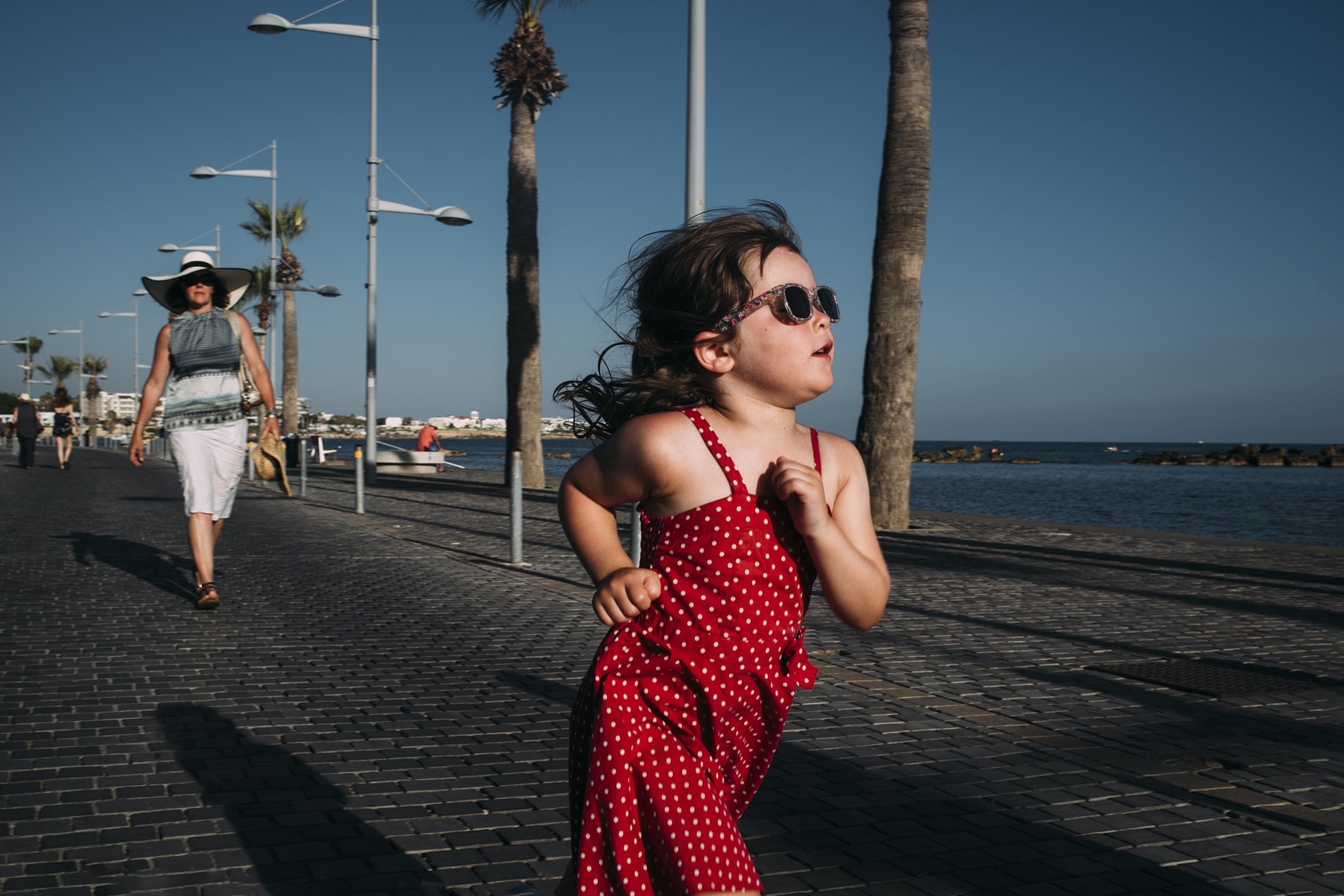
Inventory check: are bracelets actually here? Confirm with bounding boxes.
[264,408,277,419]
[134,421,147,426]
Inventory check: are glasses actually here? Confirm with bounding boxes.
[185,273,214,286]
[709,283,839,332]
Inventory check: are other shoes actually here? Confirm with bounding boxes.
[62,460,70,468]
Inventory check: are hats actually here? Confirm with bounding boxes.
[252,431,291,497]
[17,393,32,401]
[140,251,253,314]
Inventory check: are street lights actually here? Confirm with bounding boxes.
[189,139,343,401]
[0,331,53,397]
[48,320,109,410]
[246,0,473,488]
[98,301,139,406]
[157,224,221,267]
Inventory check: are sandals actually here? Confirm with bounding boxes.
[197,581,219,607]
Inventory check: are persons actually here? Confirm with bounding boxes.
[129,251,280,606]
[557,200,893,896]
[417,422,446,473]
[0,387,81,471]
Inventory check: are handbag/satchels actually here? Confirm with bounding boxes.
[232,311,263,407]
[34,419,44,433]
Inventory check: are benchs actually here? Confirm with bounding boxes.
[375,449,444,475]
